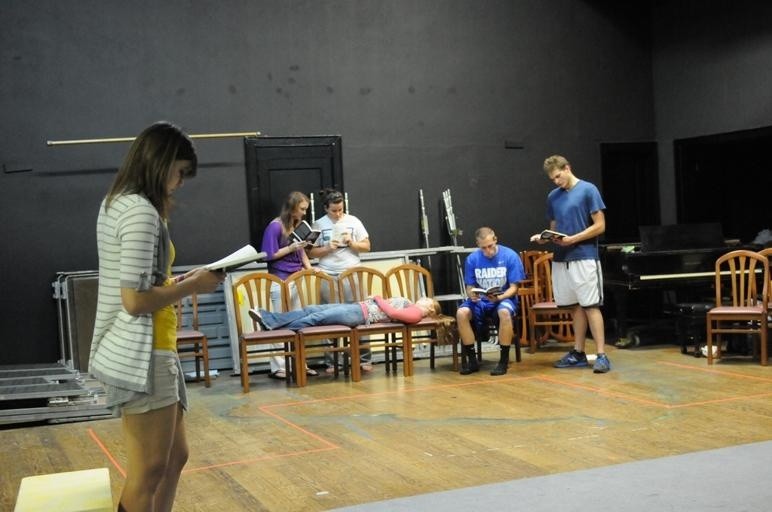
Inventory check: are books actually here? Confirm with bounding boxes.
[530,227,566,244]
[287,219,321,253]
[202,242,269,273]
[321,226,355,248]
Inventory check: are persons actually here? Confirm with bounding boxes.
[306,184,374,375]
[262,190,318,379]
[470,284,503,300]
[86,119,226,512]
[248,293,460,347]
[454,226,526,376]
[539,152,611,373]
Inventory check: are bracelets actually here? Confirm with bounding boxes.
[174,276,177,284]
[179,274,185,282]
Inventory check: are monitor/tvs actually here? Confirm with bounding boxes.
[673,126,771,242]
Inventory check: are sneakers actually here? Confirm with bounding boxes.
[492,364,509,376]
[459,363,479,375]
[553,351,588,367]
[592,356,609,373]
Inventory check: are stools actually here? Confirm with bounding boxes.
[669,300,753,358]
[14,466,116,512]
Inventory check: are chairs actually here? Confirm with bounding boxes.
[706,250,768,365]
[749,246,771,359]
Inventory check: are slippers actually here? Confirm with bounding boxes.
[269,367,320,380]
